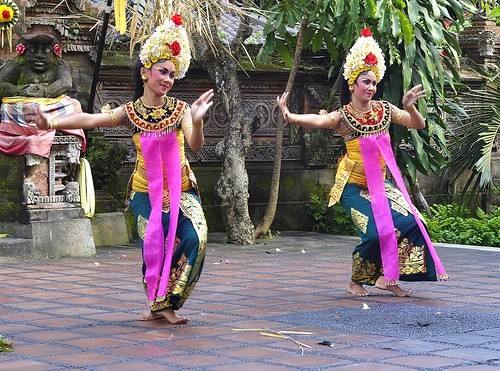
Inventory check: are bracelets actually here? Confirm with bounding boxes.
[191,120,203,128]
[405,105,417,114]
[292,113,301,125]
[49,119,58,129]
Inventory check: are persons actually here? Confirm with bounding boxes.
[25,53,214,324]
[277,62,449,297]
[0,33,72,98]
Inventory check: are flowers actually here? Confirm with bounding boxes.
[54,44,61,55]
[16,44,25,55]
[139,14,192,80]
[343,28,386,90]
[0,4,14,23]
